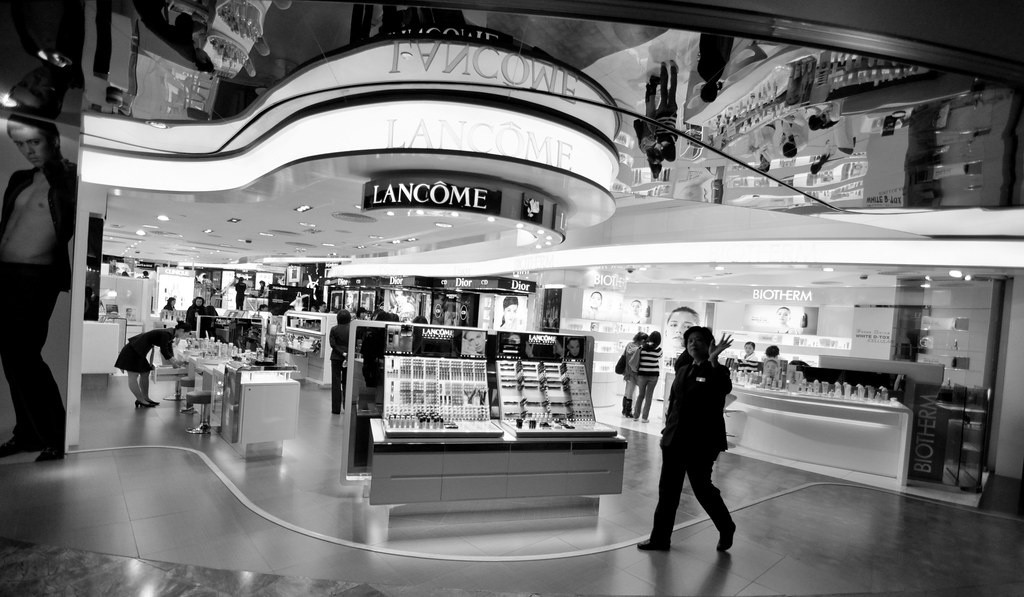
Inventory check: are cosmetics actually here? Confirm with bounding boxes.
[384,353,595,431]
[729,366,897,404]
[183,336,250,361]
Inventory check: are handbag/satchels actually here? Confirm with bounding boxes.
[628,346,643,373]
[614,351,626,374]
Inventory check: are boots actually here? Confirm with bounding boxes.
[622,397,627,415]
[626,399,634,418]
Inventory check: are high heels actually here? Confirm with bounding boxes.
[135,398,159,407]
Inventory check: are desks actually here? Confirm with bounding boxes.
[728,375,913,486]
[171,344,260,433]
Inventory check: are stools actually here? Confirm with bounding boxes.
[186,390,213,434]
[164,360,188,401]
[178,376,196,415]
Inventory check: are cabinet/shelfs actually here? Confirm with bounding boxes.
[369,323,629,513]
[286,309,340,386]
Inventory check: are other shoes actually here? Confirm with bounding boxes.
[634,418,638,422]
[716,523,736,551]
[638,540,670,551]
[35,447,64,462]
[0,438,41,457]
[642,419,650,422]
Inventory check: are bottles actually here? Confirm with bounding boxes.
[188,336,242,363]
[727,370,898,404]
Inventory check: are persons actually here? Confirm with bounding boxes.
[637,326,734,553]
[186,295,219,340]
[114,297,189,409]
[120,270,149,280]
[638,59,680,179]
[566,338,580,359]
[629,300,642,324]
[767,307,799,334]
[498,297,525,332]
[1,114,78,465]
[462,330,485,354]
[584,292,606,321]
[197,274,268,311]
[310,301,327,313]
[387,290,415,322]
[696,32,735,103]
[665,306,700,355]
[748,100,856,172]
[329,300,428,414]
[741,342,782,375]
[621,331,663,422]
